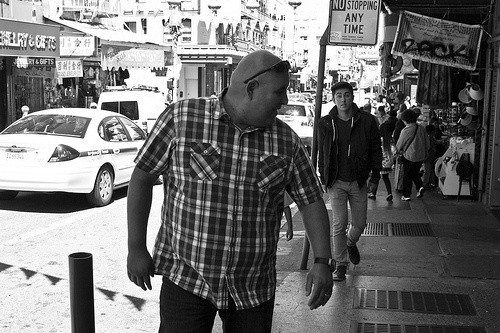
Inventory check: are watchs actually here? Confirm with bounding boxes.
[313,256,337,273]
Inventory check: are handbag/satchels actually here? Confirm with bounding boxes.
[398,153,404,164]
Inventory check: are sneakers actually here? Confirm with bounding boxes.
[401,196,411,200]
[416,186,424,198]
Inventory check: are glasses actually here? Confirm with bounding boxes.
[244,60,291,84]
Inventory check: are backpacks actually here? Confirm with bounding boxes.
[381,152,392,171]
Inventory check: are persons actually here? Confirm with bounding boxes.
[19,105,29,118]
[392,108,431,202]
[210,92,217,98]
[315,80,385,282]
[368,122,398,202]
[124,49,339,333]
[362,92,411,146]
[283,190,295,242]
[89,103,98,109]
[423,124,439,189]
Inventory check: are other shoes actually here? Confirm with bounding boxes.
[333,265,346,281]
[368,193,376,199]
[348,244,361,265]
[386,194,393,201]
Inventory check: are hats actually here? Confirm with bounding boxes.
[458,85,484,127]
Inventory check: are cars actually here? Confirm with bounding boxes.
[276,96,317,152]
[0,107,158,208]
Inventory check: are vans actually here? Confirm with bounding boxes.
[96,85,168,135]
